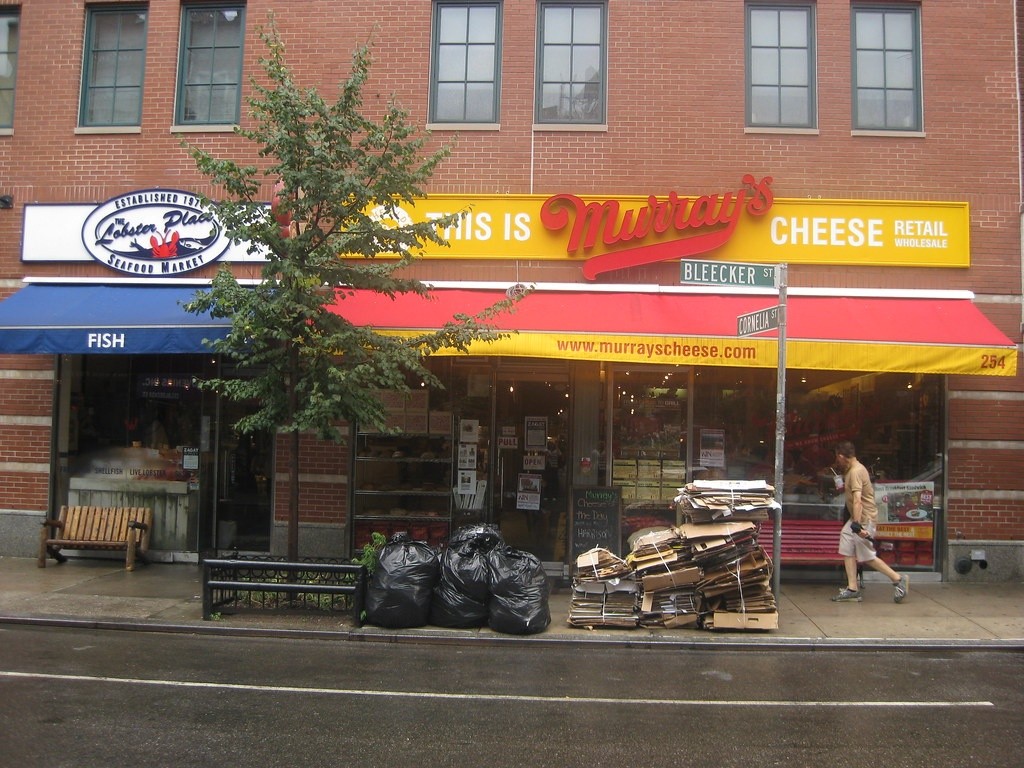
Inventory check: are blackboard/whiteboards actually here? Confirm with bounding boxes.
[568,486,622,575]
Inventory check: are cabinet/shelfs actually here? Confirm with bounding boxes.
[346,414,455,554]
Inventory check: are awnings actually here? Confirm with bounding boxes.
[315,281,1017,374]
[1,283,264,352]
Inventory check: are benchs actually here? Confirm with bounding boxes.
[37,506,154,572]
[755,519,866,587]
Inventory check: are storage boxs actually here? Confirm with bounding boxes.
[358,388,453,434]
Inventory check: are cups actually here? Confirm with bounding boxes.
[834,475,844,489]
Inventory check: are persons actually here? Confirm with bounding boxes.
[833,441,909,602]
[544,441,564,501]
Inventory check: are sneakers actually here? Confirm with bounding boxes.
[831,586,862,602]
[894,574,909,603]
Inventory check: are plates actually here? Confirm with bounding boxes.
[906,509,927,519]
